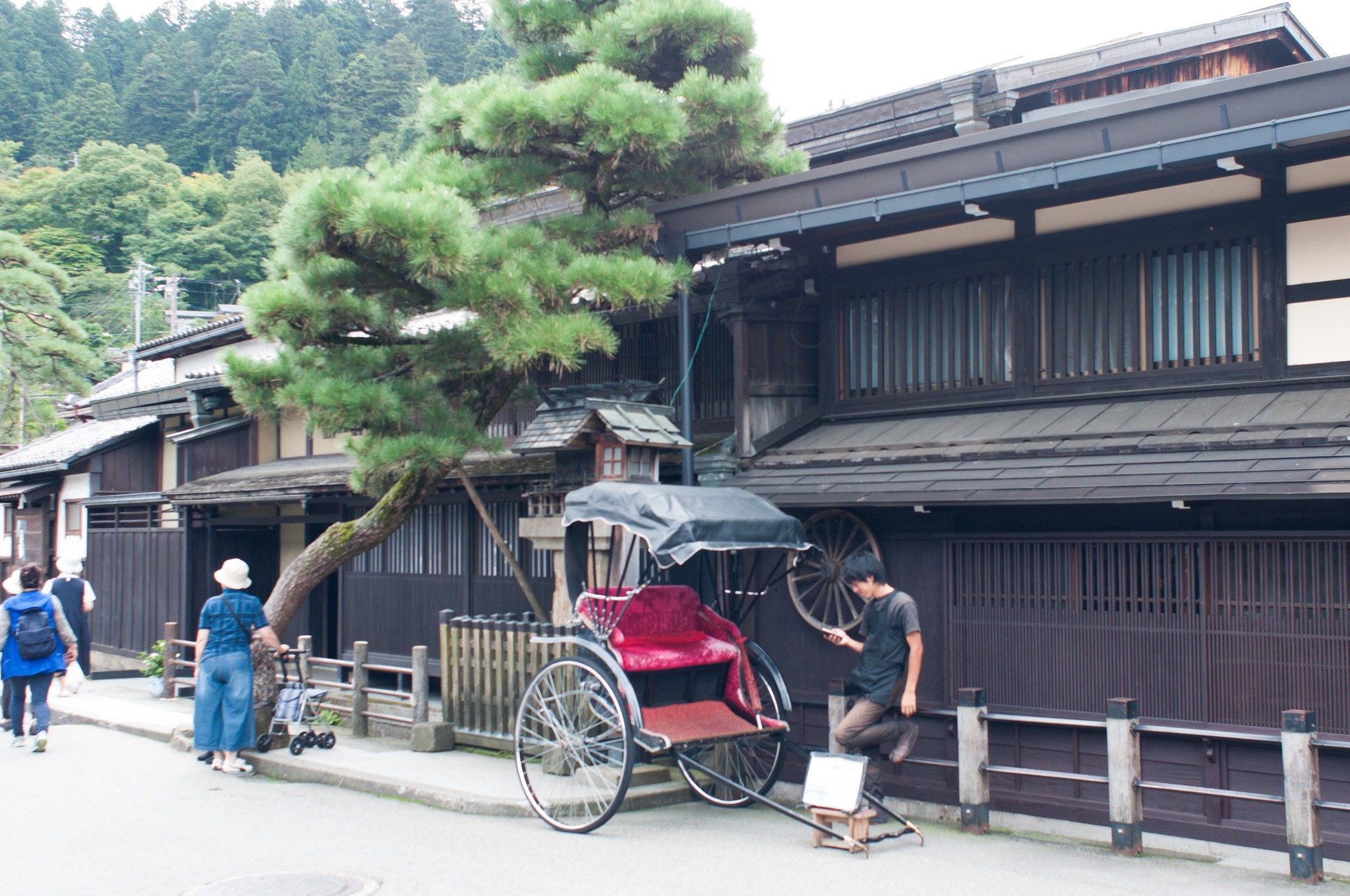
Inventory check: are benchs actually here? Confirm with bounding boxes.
[580,586,738,671]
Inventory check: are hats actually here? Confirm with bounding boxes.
[56,552,83,574]
[2,569,25,594]
[214,559,252,590]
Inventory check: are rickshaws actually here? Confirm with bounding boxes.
[514,480,926,860]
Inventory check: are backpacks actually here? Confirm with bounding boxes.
[10,607,58,659]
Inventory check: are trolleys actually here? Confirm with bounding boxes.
[255,646,339,754]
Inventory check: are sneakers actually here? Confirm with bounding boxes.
[869,808,887,824]
[32,729,48,752]
[11,735,26,747]
[212,756,245,770]
[888,722,919,762]
[223,760,253,773]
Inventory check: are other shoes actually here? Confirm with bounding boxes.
[55,687,70,696]
[205,755,214,764]
[197,749,213,760]
[0,717,13,731]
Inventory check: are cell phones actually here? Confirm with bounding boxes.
[820,627,843,641]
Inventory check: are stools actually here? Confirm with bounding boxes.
[811,807,876,853]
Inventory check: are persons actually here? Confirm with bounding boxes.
[0,563,79,753]
[825,549,923,823]
[193,558,290,772]
[41,552,97,681]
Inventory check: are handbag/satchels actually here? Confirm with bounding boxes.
[66,659,85,693]
